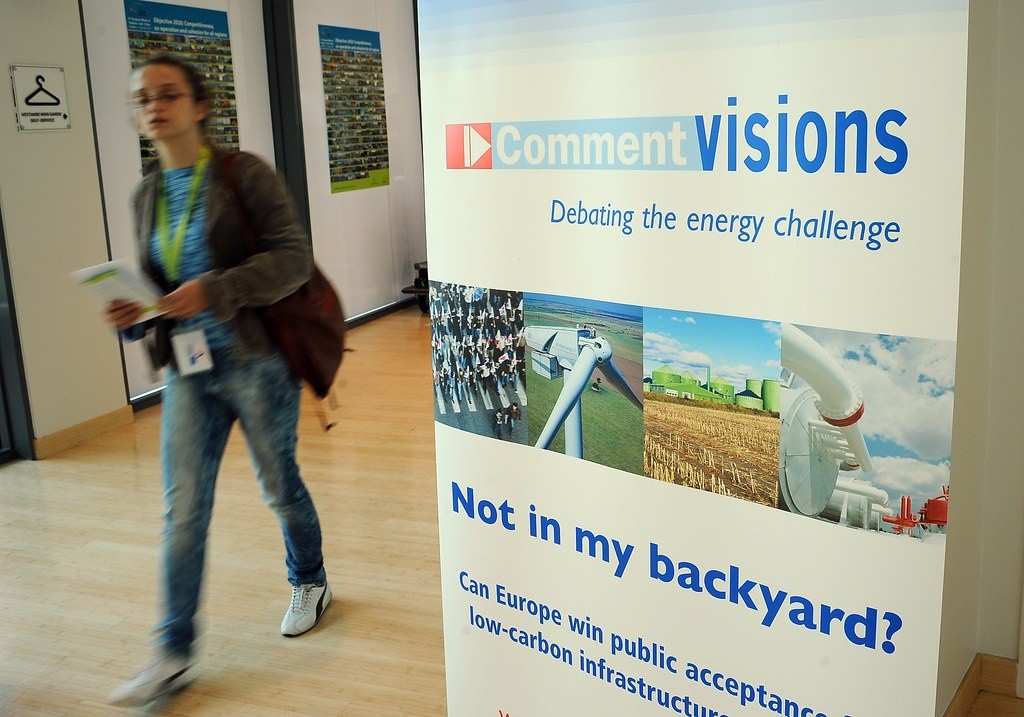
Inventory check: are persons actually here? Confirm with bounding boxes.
[493,402,522,439]
[99,53,333,707]
[429,281,526,404]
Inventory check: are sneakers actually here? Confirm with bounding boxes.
[109,653,199,707]
[280,581,332,636]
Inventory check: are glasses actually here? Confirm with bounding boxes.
[125,89,196,108]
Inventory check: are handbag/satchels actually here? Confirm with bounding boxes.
[261,266,346,398]
